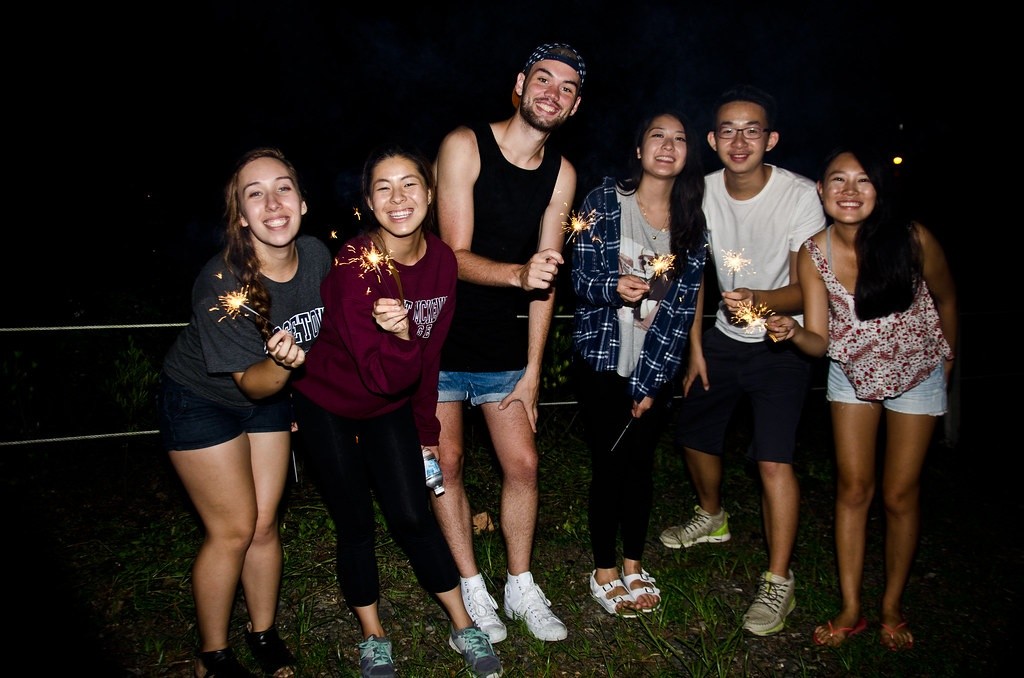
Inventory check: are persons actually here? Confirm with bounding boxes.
[154,42,587,678]
[571,84,956,652]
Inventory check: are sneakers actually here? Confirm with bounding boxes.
[460,573,507,644]
[505,570,567,642]
[742,568,796,636]
[449,622,503,678]
[359,634,394,677]
[659,505,731,548]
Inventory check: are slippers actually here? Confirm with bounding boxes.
[879,622,913,652]
[813,617,867,647]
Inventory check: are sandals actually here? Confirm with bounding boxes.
[246,626,298,677]
[621,566,661,613]
[590,569,642,618]
[193,647,253,678]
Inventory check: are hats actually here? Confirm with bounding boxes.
[511,43,586,109]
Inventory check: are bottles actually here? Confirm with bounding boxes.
[422,449,444,495]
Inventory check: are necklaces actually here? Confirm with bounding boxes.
[637,190,669,240]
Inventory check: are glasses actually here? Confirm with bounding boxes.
[715,127,770,139]
